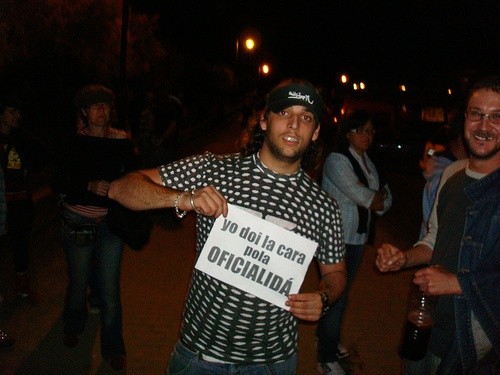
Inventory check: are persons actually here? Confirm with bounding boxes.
[0,86,257,371]
[374,79,500,375]
[312,109,393,375]
[107,79,349,375]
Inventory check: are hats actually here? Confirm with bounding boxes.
[266,79,323,123]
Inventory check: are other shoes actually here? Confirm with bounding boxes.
[0,329,15,347]
[111,353,127,370]
[63,333,79,348]
[16,274,30,297]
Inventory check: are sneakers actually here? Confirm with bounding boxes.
[336,343,350,358]
[316,361,346,375]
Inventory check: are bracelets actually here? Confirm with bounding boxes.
[314,287,330,311]
[381,188,387,199]
[174,193,190,219]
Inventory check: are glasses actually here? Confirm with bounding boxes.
[88,103,111,110]
[350,127,375,136]
[464,107,500,124]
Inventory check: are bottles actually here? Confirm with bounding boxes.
[397,261,436,361]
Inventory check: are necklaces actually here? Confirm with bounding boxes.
[81,128,108,138]
